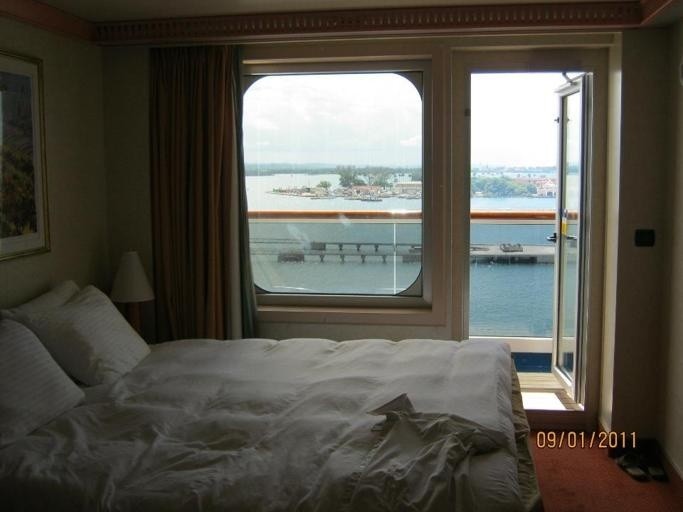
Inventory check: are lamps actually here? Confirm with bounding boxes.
[110,251,157,335]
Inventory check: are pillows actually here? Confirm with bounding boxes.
[0,279,150,450]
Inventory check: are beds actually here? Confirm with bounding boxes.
[1,339,546,512]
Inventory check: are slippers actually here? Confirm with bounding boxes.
[639,440,667,480]
[615,454,647,481]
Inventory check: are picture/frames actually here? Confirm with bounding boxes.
[0,47,50,262]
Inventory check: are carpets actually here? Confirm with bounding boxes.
[522,392,567,413]
